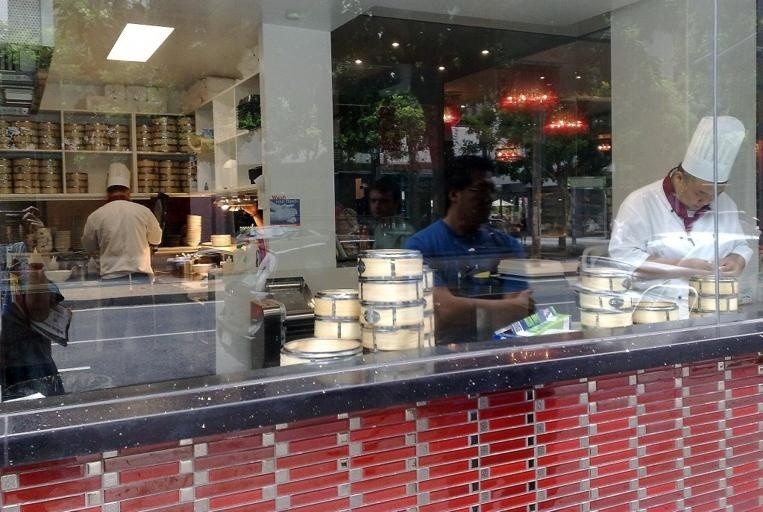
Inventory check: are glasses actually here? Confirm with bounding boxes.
[466,187,501,206]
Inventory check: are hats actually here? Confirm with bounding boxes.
[681,115,746,185]
[105,161,131,190]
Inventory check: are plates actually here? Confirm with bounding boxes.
[52,230,85,252]
[184,213,232,246]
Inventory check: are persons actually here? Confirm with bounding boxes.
[606,115,754,321]
[368,177,416,251]
[80,161,163,350]
[0,205,65,401]
[405,154,536,346]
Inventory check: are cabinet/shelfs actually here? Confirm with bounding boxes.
[194,71,261,190]
[0,108,196,203]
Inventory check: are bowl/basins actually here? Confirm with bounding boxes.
[43,270,71,282]
